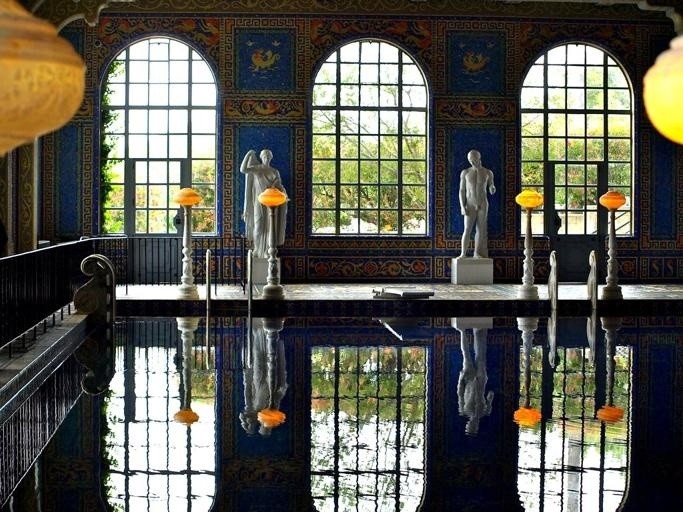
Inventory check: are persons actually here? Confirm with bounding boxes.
[252,329,289,436]
[240,148,291,259]
[456,329,495,440]
[456,150,497,258]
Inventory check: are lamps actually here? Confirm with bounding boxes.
[174,316,201,424]
[599,191,627,299]
[513,317,539,426]
[644,36,683,145]
[258,188,287,299]
[173,188,203,294]
[515,188,544,298]
[0,0,88,158]
[257,318,287,428]
[596,316,625,426]
